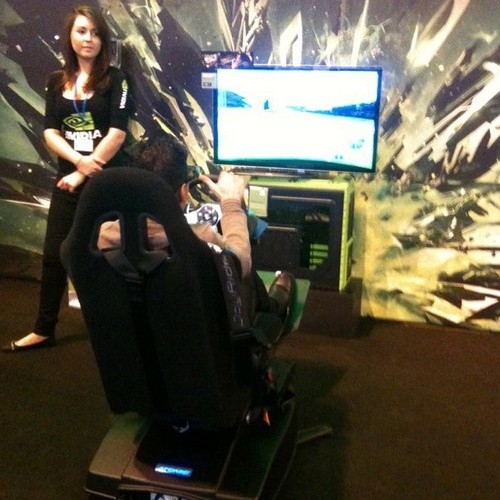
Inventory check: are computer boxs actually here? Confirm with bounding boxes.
[243,176,356,294]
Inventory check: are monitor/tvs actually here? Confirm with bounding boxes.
[213,64,382,173]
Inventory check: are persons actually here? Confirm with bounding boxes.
[2,4,133,354]
[94,135,298,335]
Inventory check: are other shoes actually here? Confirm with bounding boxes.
[1,335,55,352]
[267,273,296,315]
